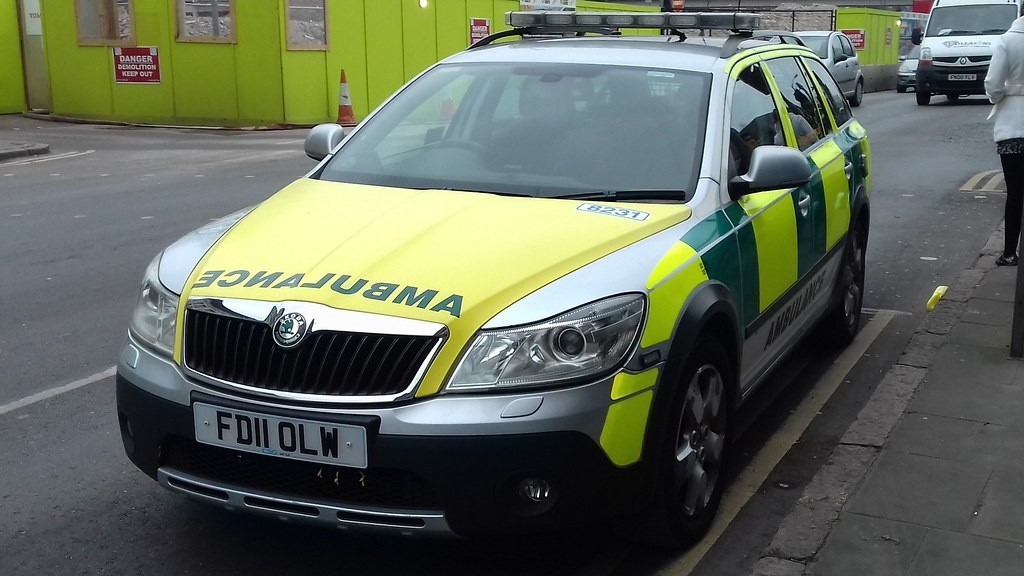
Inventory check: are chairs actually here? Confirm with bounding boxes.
[590,72,658,174]
[496,79,587,179]
[634,85,738,185]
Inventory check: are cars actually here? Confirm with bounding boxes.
[770,32,865,108]
[895,32,921,93]
[916,0,1024,106]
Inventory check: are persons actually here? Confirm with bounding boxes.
[983,5,1024,266]
[636,80,737,179]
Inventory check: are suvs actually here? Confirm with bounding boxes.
[105,4,875,549]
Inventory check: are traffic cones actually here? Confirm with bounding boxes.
[331,68,358,126]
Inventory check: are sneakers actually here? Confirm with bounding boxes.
[997,254,1020,265]
